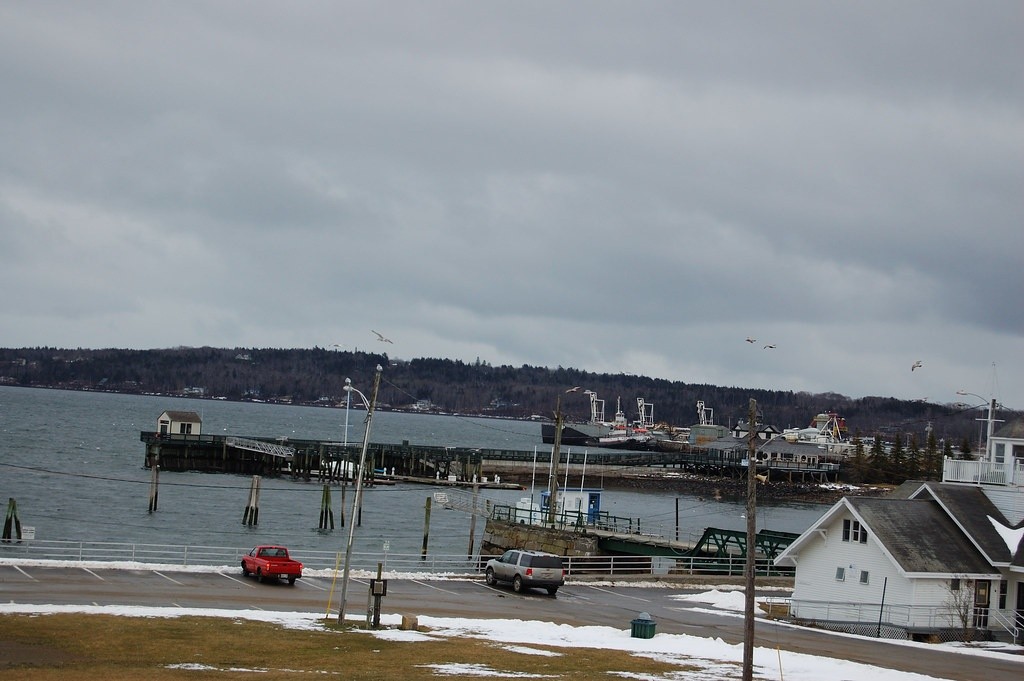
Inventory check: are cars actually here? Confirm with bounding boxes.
[485,550,565,595]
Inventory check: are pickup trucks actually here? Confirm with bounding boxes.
[241,546,303,585]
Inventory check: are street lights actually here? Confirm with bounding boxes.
[345,377,351,446]
[742,398,820,681]
[338,364,383,626]
[957,390,993,459]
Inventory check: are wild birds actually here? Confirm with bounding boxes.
[764,344,776,349]
[912,361,923,371]
[565,387,580,393]
[329,343,342,348]
[746,337,756,343]
[371,330,393,344]
